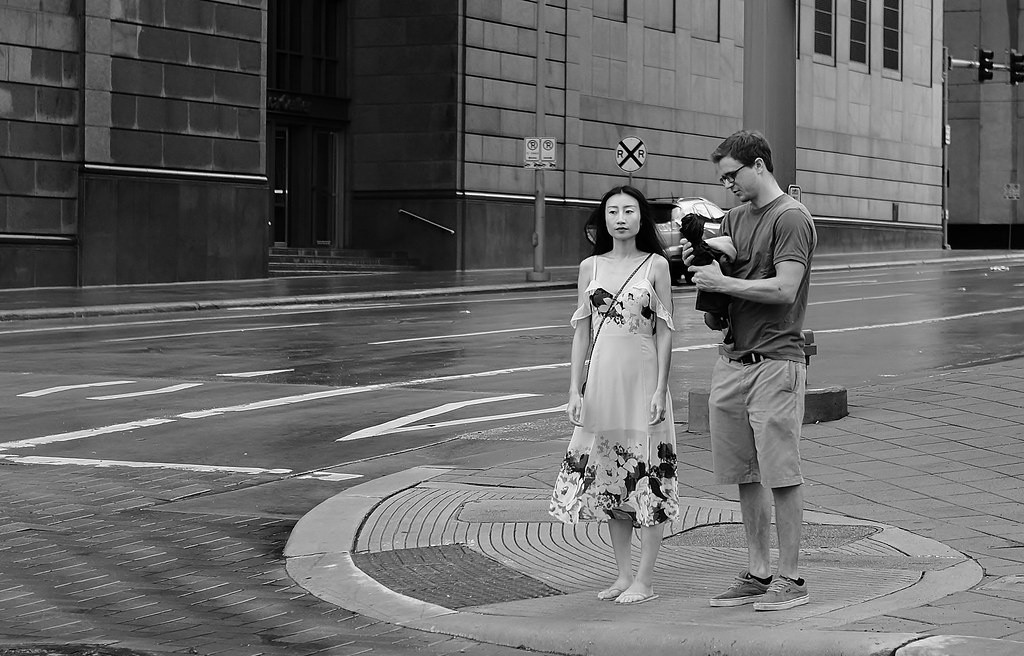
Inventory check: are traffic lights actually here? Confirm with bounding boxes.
[979,49,993,84]
[1010,49,1024,85]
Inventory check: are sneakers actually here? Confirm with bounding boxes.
[753,573,810,612]
[709,570,765,608]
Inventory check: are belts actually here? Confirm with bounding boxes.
[722,352,766,365]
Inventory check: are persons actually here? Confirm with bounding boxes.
[548,185,679,605]
[680,128,817,609]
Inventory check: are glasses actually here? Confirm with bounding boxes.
[719,164,748,184]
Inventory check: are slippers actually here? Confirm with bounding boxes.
[597,586,622,602]
[615,592,660,606]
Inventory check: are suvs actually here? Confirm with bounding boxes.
[648,194,726,283]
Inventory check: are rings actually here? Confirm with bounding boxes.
[660,416,665,421]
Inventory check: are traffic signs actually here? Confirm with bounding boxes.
[523,137,556,170]
